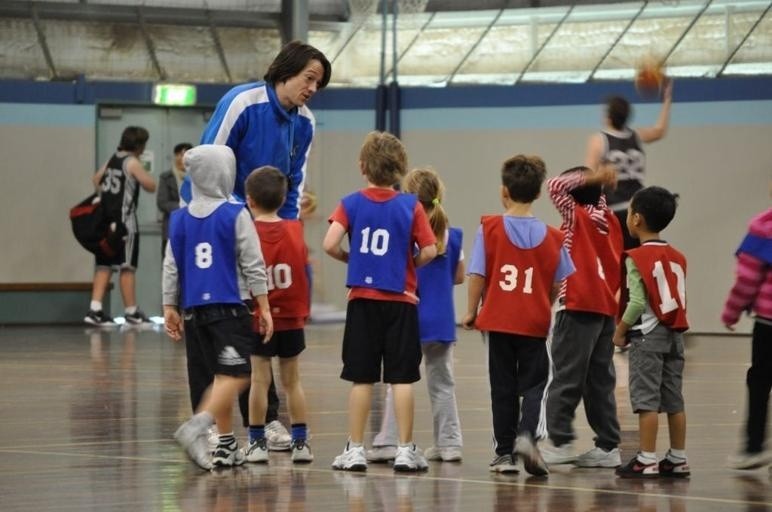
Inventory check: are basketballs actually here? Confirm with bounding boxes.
[637,66,664,94]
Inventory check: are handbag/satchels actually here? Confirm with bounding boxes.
[69,190,129,266]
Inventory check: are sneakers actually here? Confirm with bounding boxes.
[512,431,550,476]
[486,451,520,472]
[614,457,660,479]
[723,447,772,469]
[242,438,269,462]
[573,447,622,468]
[330,446,369,472]
[424,442,463,462]
[206,421,219,452]
[120,306,156,330]
[211,440,246,467]
[290,438,313,462]
[83,308,117,326]
[365,444,397,462]
[661,458,692,477]
[540,441,579,464]
[392,443,429,471]
[172,414,212,470]
[263,420,293,451]
